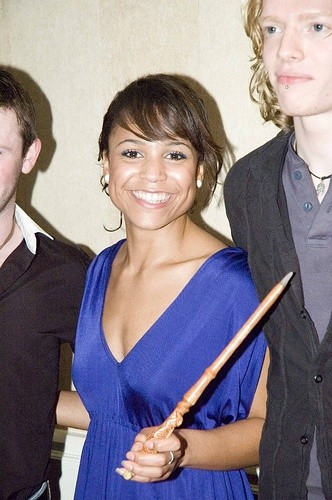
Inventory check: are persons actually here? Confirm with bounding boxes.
[223,0,332,500]
[0,64,92,500]
[53,73,293,500]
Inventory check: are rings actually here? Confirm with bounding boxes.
[169,450,174,465]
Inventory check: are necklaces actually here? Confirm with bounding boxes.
[295,142,332,196]
